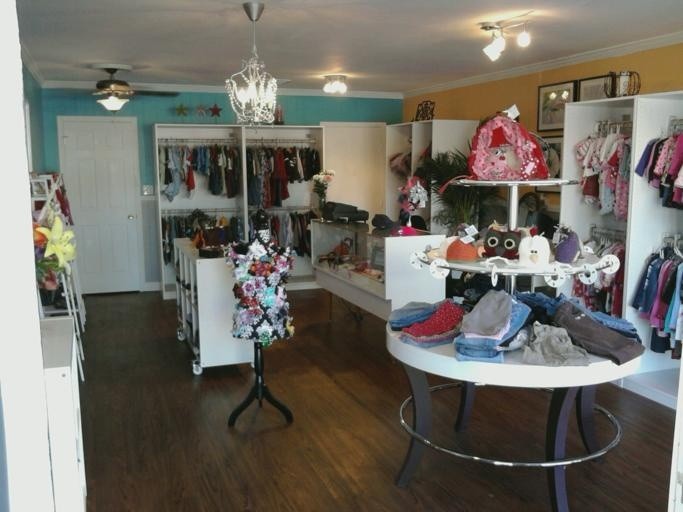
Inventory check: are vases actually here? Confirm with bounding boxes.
[310,192,327,223]
[39,288,57,306]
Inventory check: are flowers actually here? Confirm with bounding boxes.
[32,216,76,291]
[312,169,335,211]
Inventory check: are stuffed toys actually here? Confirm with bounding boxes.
[439,223,583,266]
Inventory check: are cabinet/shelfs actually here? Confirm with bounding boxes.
[310,218,435,365]
[30,172,87,381]
[385,179,640,512]
[386,119,481,235]
[39,316,87,512]
[244,125,325,291]
[556,90,683,410]
[174,238,256,375]
[152,123,247,299]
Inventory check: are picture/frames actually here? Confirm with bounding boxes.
[535,135,562,194]
[577,72,616,101]
[537,80,577,131]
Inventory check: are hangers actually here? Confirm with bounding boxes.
[582,226,627,256]
[158,137,239,148]
[246,136,318,154]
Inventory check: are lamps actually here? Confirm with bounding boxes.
[92,63,133,113]
[323,75,348,94]
[479,21,530,62]
[224,1,278,124]
